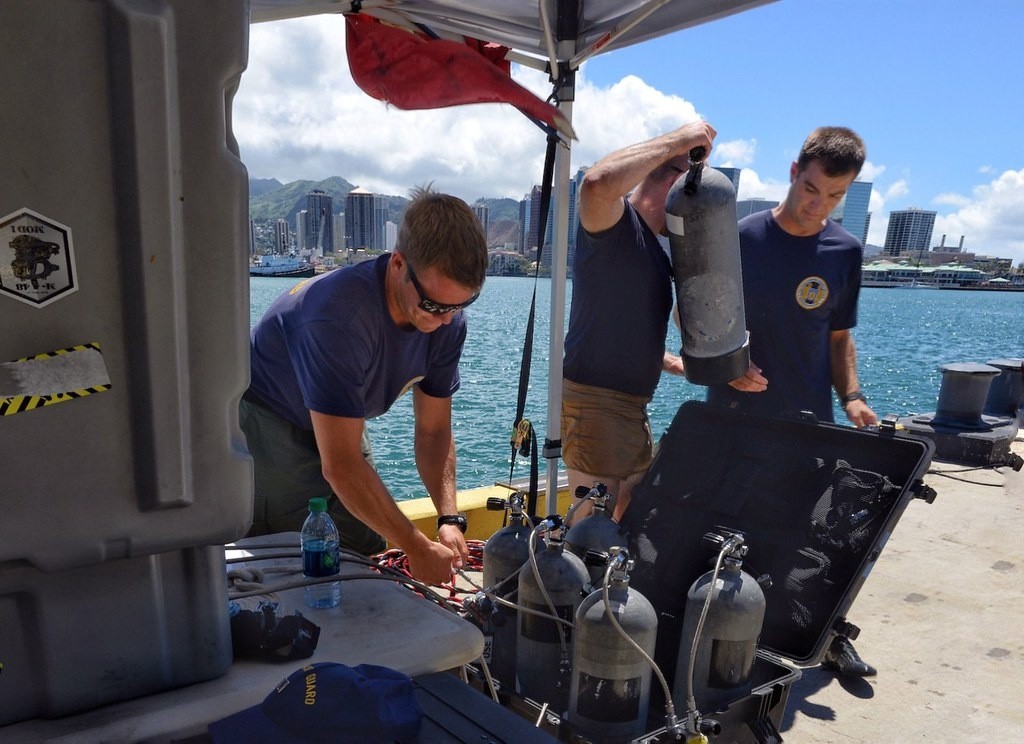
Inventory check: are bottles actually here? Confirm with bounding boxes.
[299,498,343,610]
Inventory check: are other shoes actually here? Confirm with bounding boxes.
[822,635,877,677]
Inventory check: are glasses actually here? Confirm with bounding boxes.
[406,260,482,315]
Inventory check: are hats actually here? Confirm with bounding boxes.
[206,662,423,744]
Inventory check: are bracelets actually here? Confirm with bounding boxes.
[438,515,467,534]
[841,392,866,411]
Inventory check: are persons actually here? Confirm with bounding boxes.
[253,256,260,267]
[672,126,876,428]
[240,193,489,585]
[562,120,718,530]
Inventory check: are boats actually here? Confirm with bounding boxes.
[250,248,315,277]
